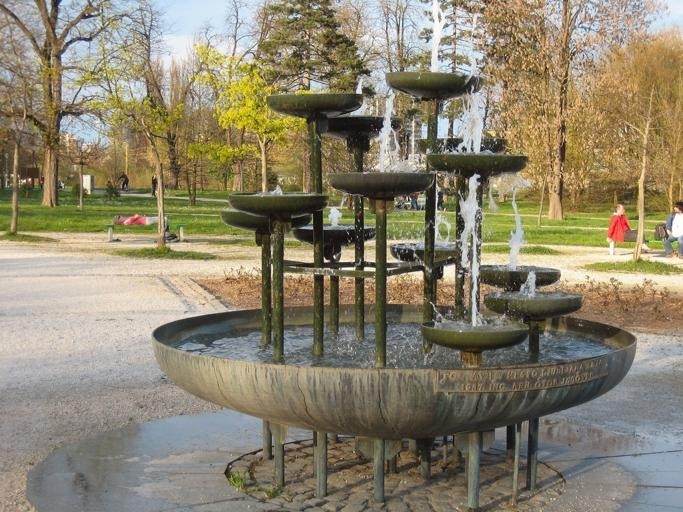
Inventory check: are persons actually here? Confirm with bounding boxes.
[395,195,406,209]
[661,201,682,258]
[112,213,168,226]
[150,175,157,197]
[408,192,418,210]
[605,203,652,251]
[435,190,446,212]
[117,171,129,192]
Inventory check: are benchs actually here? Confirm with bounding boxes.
[106,222,186,242]
[609,238,679,255]
